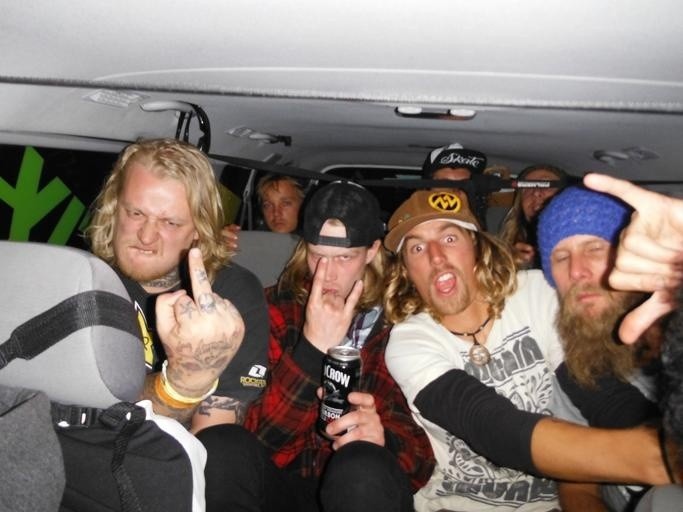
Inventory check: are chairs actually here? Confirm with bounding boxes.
[222,232,299,288]
[1,236,207,512]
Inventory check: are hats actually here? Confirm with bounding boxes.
[422,142,489,179]
[383,189,482,262]
[301,179,386,249]
[535,186,634,287]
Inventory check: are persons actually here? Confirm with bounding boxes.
[49,138,437,512]
[384,143,683,512]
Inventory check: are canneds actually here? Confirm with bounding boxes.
[317,345,363,440]
